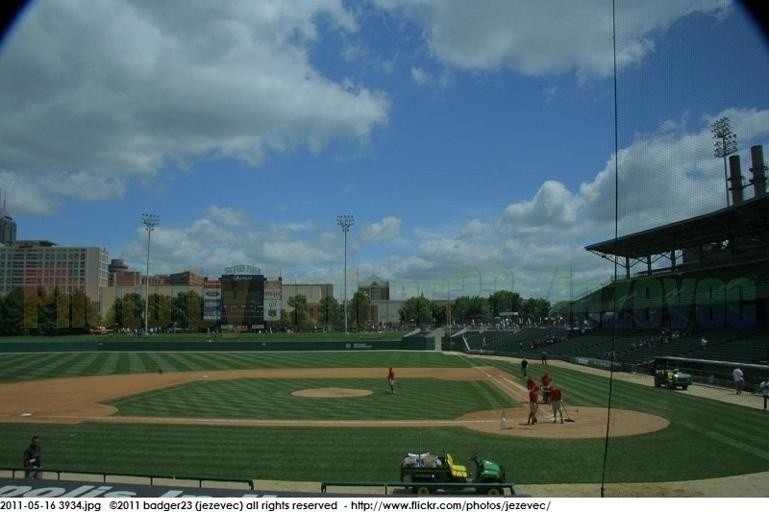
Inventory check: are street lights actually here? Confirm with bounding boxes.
[335,213,357,336]
[139,211,164,335]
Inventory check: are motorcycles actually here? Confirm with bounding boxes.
[401,451,506,496]
[654,368,692,391]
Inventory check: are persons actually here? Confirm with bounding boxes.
[760,377,769,411]
[540,349,548,366]
[349,315,397,340]
[630,327,687,350]
[521,357,528,377]
[516,334,570,357]
[732,366,745,394]
[89,325,163,338]
[387,367,395,393]
[696,334,712,356]
[524,371,564,425]
[451,313,559,329]
[24,436,42,479]
